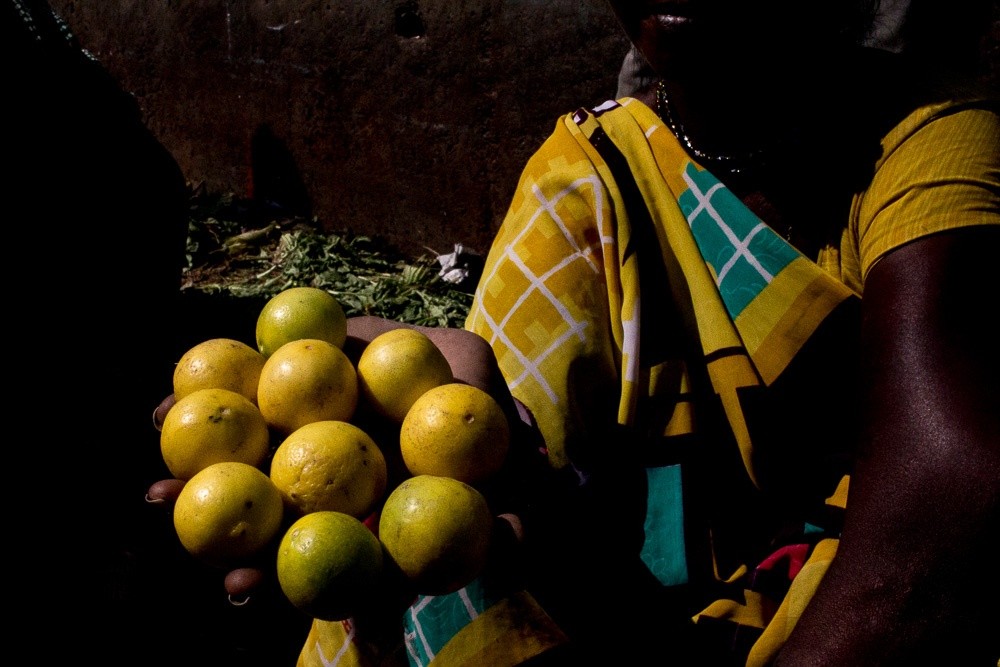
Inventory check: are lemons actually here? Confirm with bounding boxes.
[159,287,508,622]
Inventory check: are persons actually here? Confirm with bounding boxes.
[145,0,1000,667]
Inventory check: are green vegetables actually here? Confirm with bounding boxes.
[173,186,483,329]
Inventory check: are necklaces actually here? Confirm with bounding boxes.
[653,77,830,178]
